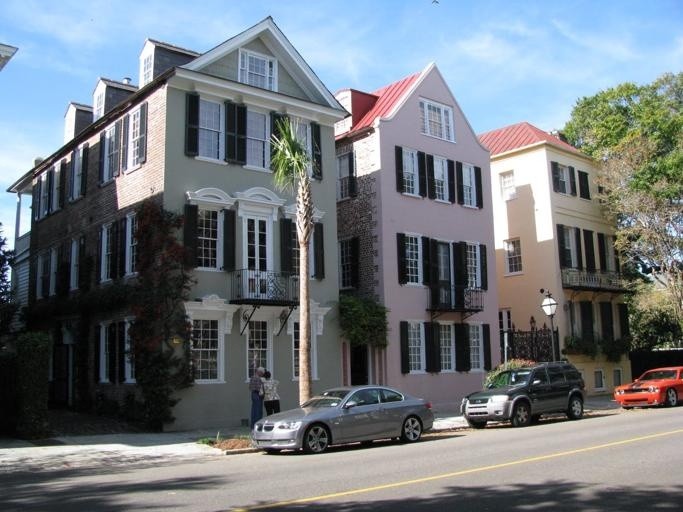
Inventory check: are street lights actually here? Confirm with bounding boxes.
[539,289,562,363]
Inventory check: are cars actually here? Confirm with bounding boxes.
[613,367,683,408]
[252,385,431,453]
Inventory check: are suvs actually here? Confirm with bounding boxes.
[460,362,588,430]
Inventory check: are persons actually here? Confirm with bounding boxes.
[257,370,279,416]
[248,367,265,430]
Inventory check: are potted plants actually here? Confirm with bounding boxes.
[564,334,580,354]
